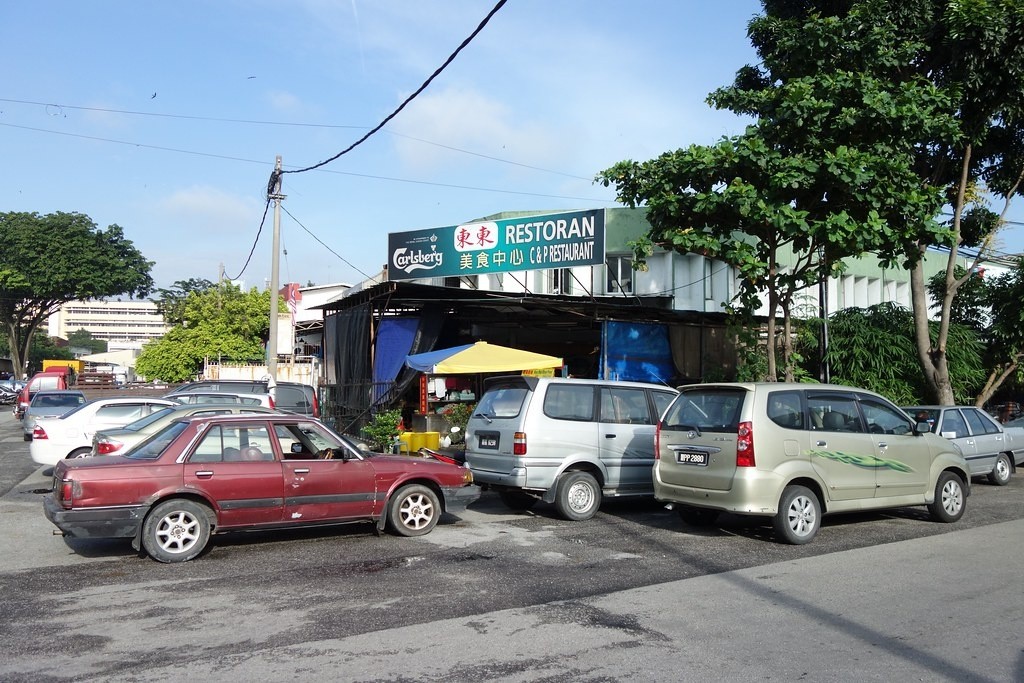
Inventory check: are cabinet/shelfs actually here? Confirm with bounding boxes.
[428,374,477,415]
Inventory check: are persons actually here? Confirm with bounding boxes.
[916,411,928,421]
[1002,402,1016,423]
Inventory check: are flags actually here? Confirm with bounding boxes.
[289,288,296,326]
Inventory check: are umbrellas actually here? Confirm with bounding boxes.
[405,339,562,400]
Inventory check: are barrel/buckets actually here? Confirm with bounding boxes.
[399,432,440,452]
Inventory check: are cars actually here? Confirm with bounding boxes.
[900,405,1024,485]
[30,396,474,564]
[21,391,88,442]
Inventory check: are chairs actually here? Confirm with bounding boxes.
[222,447,240,461]
[777,409,795,427]
[944,419,963,437]
[240,447,264,461]
[724,409,735,424]
[822,412,851,432]
[629,407,646,424]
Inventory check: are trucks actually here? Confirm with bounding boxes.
[11,364,189,419]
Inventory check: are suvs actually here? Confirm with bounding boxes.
[463,374,710,521]
[168,380,320,419]
[651,381,972,545]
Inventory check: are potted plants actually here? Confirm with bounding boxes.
[360,410,403,454]
[65,397,81,407]
[40,397,53,406]
